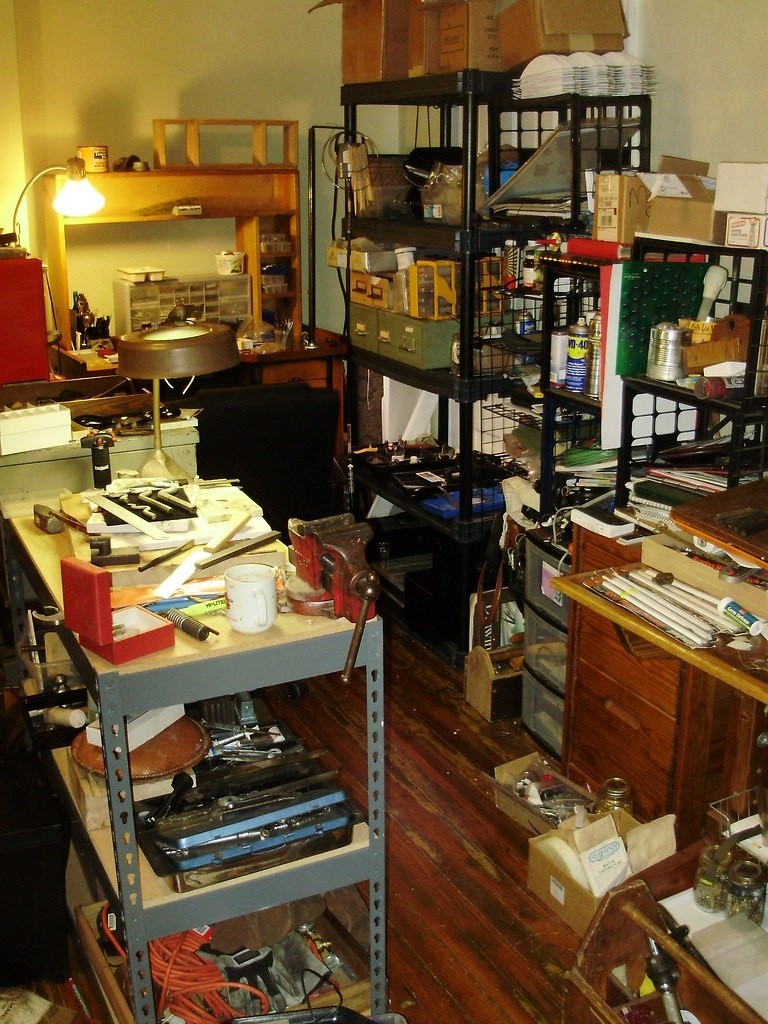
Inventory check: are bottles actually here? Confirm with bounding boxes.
[501,240,520,289]
[565,318,590,392]
[523,250,534,289]
[595,778,634,848]
[515,308,534,364]
[584,310,602,397]
[524,241,537,262]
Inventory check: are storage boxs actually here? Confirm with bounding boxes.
[307,0,631,83]
[350,270,395,308]
[324,244,398,273]
[376,308,522,370]
[61,557,175,666]
[420,186,490,227]
[74,897,373,1024]
[527,809,677,939]
[349,301,381,352]
[402,569,461,643]
[481,752,599,836]
[593,174,651,243]
[1,427,198,520]
[16,629,81,693]
[636,155,728,245]
[355,185,409,219]
[86,703,185,754]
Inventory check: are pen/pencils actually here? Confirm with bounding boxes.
[96,314,108,329]
[277,318,293,331]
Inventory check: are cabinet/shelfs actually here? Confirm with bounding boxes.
[0,62,768,1024]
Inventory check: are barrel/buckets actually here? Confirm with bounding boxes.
[679,317,722,344]
[646,326,693,380]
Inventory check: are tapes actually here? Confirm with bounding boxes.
[692,377,728,401]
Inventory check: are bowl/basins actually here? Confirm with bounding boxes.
[215,252,245,275]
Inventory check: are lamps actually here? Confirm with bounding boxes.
[13,157,106,244]
[118,304,243,483]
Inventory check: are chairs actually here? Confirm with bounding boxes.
[162,382,349,546]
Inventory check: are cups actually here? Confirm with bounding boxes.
[274,330,293,349]
[224,564,277,633]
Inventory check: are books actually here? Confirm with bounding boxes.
[484,117,641,219]
